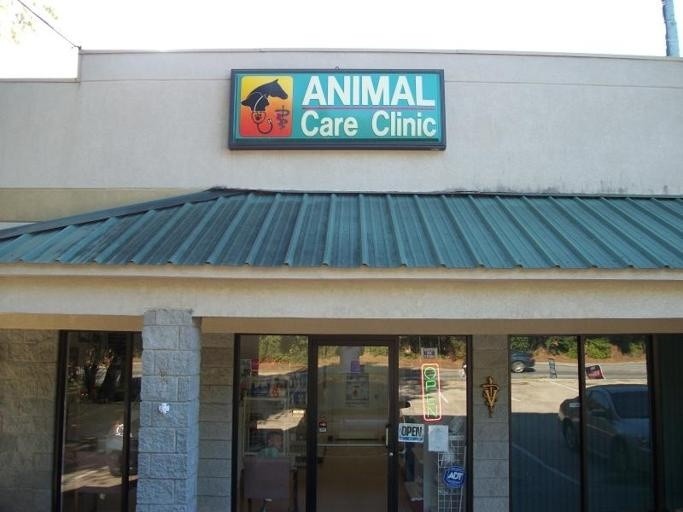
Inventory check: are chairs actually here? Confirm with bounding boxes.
[240,458,298,512]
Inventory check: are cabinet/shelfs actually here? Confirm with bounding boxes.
[242,396,291,457]
[403,415,467,512]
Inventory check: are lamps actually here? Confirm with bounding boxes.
[480,376,499,418]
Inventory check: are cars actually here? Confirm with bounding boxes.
[294,383,411,481]
[101,399,139,480]
[463,348,538,377]
[551,380,652,472]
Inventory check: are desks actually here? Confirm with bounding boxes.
[247,408,305,457]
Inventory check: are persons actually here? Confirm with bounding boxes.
[259,431,282,460]
